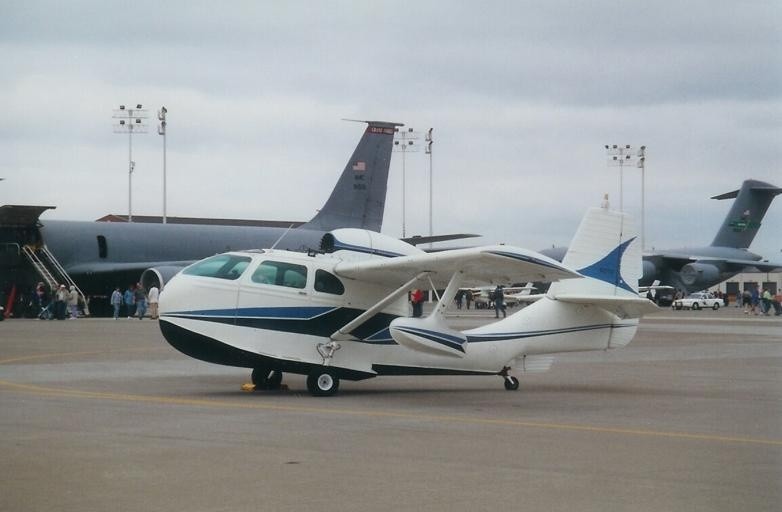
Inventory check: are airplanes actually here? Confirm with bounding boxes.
[1,120,406,322]
[160,203,647,397]
[534,179,782,312]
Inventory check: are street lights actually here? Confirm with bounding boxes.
[603,137,647,207]
[422,126,435,254]
[155,105,172,224]
[387,125,416,242]
[111,99,153,220]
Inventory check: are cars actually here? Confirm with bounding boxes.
[673,292,724,309]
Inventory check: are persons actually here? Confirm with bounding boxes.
[494,285,506,318]
[646,289,653,301]
[110,282,159,320]
[34,282,78,320]
[710,285,782,316]
[411,288,424,318]
[671,288,686,301]
[455,289,474,310]
